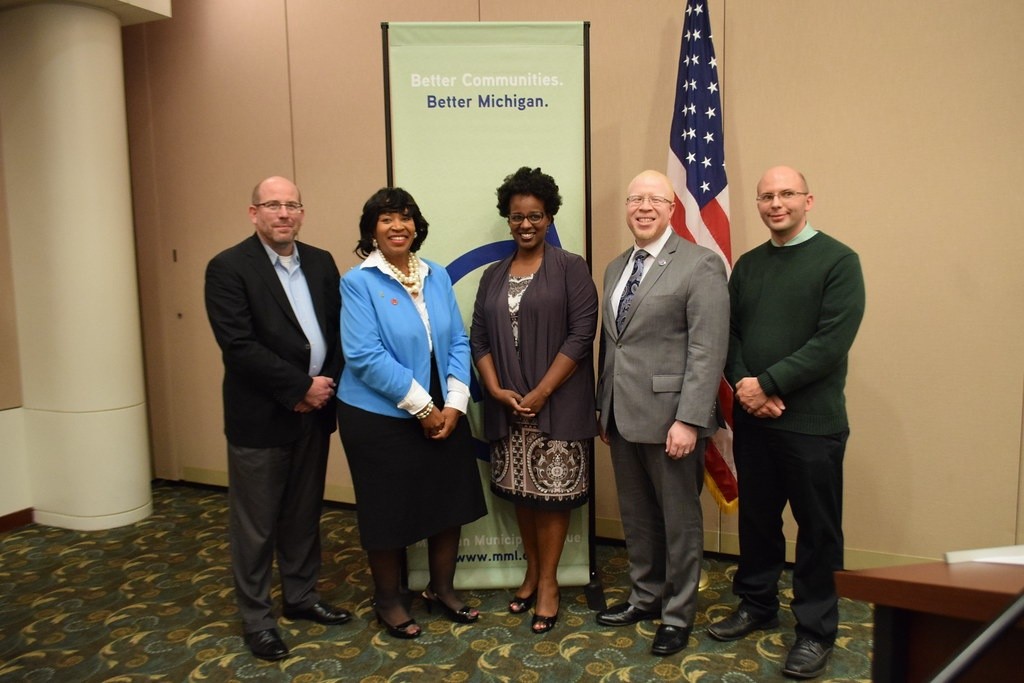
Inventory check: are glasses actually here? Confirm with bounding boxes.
[625,195,673,206]
[255,199,303,215]
[509,211,549,225]
[756,189,809,204]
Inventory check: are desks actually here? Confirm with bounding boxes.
[836,562,1024,683]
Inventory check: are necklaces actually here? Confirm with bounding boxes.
[377,247,421,295]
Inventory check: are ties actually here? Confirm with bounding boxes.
[615,250,649,335]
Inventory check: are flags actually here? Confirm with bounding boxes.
[667,0,744,513]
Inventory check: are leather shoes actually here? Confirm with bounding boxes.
[707,607,779,640]
[284,600,352,625]
[651,622,693,656]
[780,637,831,678]
[244,628,291,661]
[596,601,662,627]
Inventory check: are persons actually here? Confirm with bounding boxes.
[335,188,488,638]
[203,177,352,659]
[724,167,866,678]
[597,169,731,655]
[469,167,599,633]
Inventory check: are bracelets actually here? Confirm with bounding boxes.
[417,401,434,420]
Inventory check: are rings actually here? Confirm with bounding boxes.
[437,430,441,433]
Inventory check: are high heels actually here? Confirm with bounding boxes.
[374,599,422,639]
[532,590,561,633]
[508,580,539,615]
[420,588,481,623]
